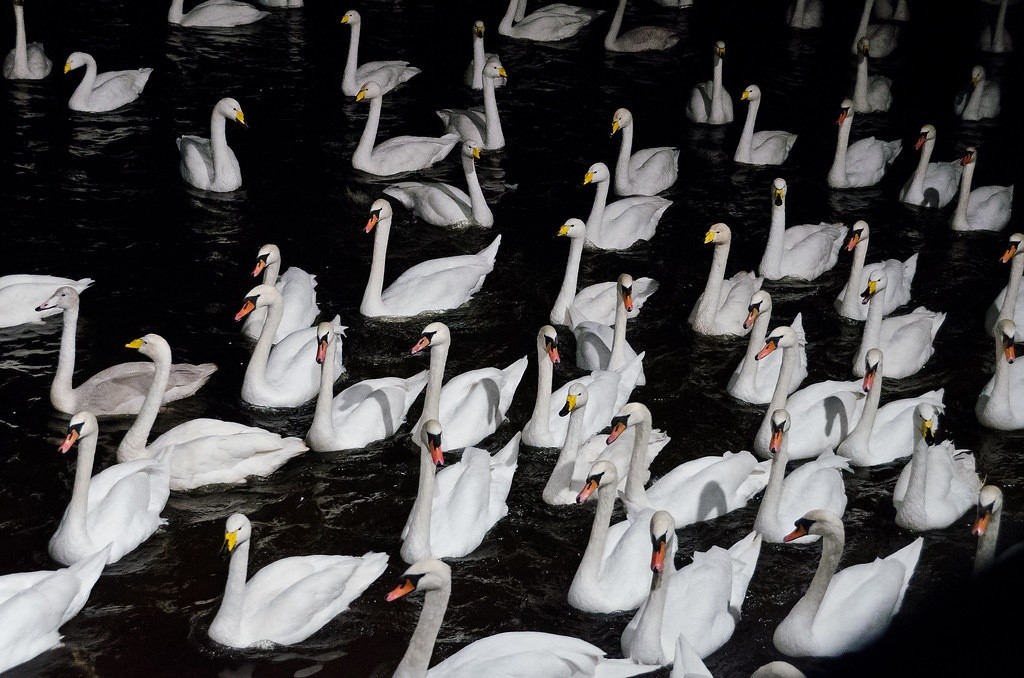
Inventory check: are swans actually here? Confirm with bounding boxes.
[0,0,1024,678]
